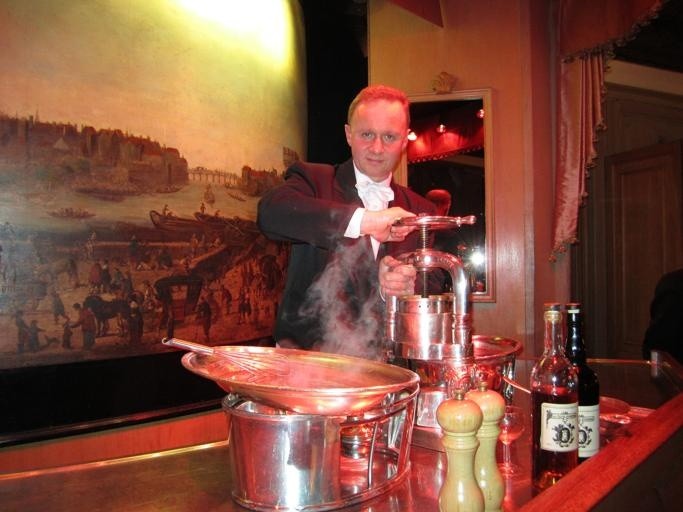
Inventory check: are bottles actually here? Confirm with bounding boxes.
[531,302,579,488]
[567,303,600,461]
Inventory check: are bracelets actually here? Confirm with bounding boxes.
[378,285,388,305]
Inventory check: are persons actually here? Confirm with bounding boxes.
[424,184,485,293]
[256,83,437,361]
[640,268,682,398]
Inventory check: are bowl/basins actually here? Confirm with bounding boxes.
[180,344,421,414]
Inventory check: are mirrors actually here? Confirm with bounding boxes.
[395,87,498,304]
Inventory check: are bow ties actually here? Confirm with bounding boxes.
[353,181,394,202]
